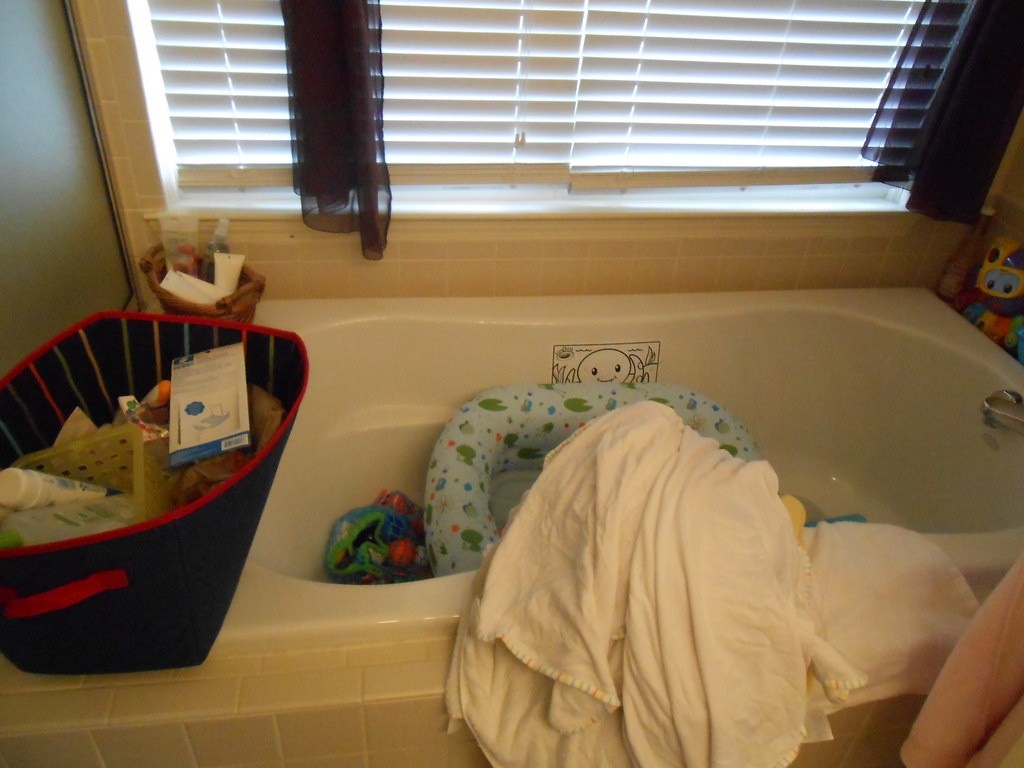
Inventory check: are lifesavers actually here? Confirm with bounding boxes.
[422,380,761,580]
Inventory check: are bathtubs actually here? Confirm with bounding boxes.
[0,286,1024,768]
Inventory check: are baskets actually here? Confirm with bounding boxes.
[139,242,265,323]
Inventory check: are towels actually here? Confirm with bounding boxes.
[441,399,1024,768]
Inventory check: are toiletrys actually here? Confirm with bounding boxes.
[0,379,171,551]
[158,211,246,305]
